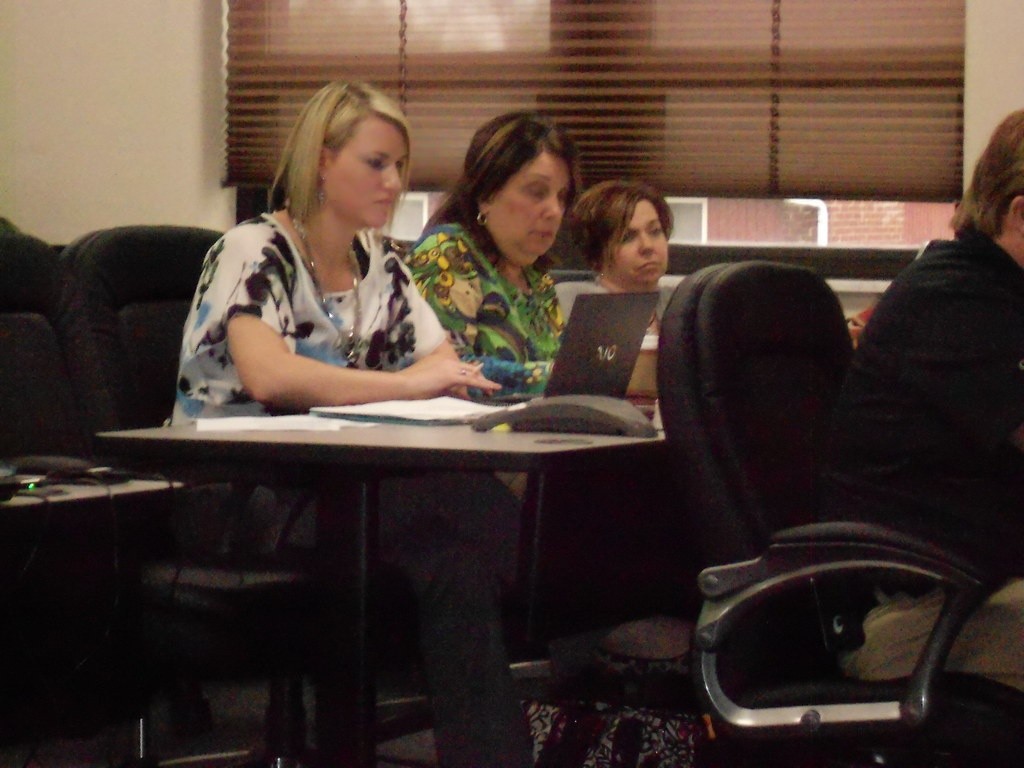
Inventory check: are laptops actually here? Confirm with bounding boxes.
[543,290,663,400]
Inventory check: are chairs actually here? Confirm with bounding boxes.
[656,262,1024,768]
[1,224,338,768]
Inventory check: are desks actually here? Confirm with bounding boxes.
[95,396,666,768]
[0,466,189,752]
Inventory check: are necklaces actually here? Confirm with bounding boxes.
[296,222,361,359]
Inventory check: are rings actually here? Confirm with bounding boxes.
[462,370,465,374]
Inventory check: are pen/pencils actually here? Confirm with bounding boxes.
[455,362,486,393]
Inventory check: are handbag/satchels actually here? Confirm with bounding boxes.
[516,693,736,768]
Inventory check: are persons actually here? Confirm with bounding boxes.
[563,178,677,398]
[173,81,502,426]
[814,109,1024,694]
[399,112,580,399]
